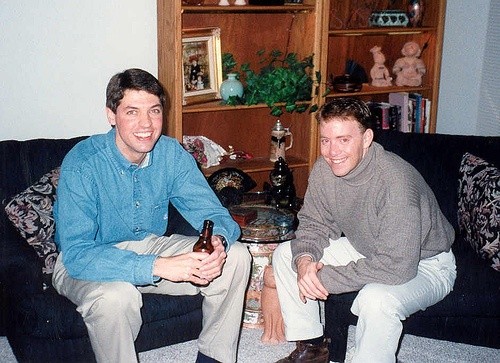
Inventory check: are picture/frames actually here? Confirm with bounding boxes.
[182,26,222,106]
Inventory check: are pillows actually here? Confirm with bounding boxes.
[5,166,60,291]
[457,152,500,273]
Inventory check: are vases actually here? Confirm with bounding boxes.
[220,74,244,101]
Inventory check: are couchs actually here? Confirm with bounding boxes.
[0,135,204,362]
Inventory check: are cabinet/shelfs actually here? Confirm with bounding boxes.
[156,0,447,198]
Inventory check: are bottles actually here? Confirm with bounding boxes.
[407,0,425,28]
[190,220,215,288]
[220,73,244,102]
[269,119,293,163]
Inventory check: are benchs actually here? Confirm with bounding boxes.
[323,130,500,363]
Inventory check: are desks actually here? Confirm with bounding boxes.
[227,202,300,333]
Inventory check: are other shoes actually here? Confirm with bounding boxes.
[275,340,329,363]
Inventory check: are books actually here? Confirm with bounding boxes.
[366,92,432,133]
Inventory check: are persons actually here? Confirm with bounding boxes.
[272,97,457,363]
[52,70,252,363]
[183,55,204,91]
[370,42,426,87]
